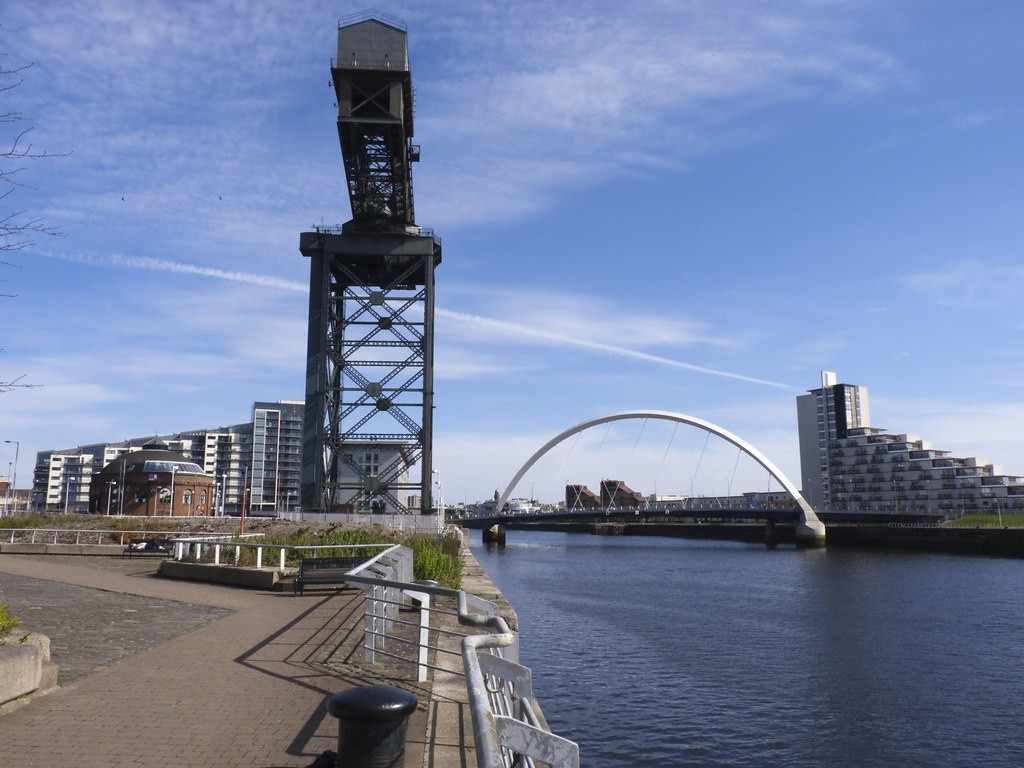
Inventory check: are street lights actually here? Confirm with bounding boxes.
[7,463,12,515]
[154,485,161,516]
[221,474,228,516]
[435,481,442,534]
[245,488,250,518]
[169,466,178,516]
[64,477,75,515]
[432,469,440,534]
[287,493,291,521]
[5,462,13,516]
[5,440,19,517]
[214,482,221,516]
[106,478,117,516]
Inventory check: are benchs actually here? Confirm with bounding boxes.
[123,539,173,559]
[292,556,382,597]
